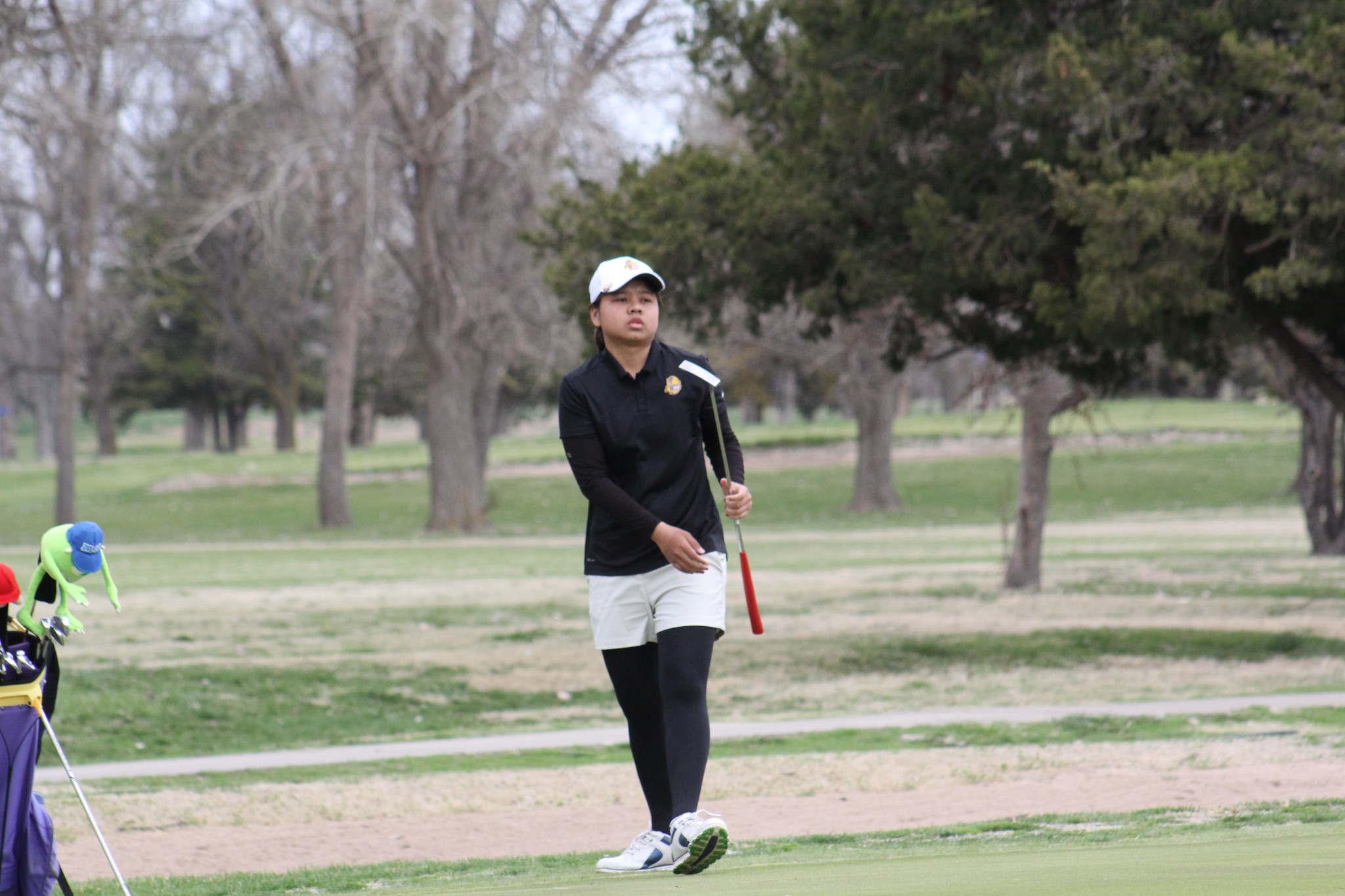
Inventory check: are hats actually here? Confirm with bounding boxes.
[0,562,20,604]
[588,255,666,303]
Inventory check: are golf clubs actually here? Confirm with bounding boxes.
[0,614,70,683]
[677,357,766,636]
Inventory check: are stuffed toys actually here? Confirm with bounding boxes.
[17,521,121,641]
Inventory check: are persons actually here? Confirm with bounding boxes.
[555,256,752,875]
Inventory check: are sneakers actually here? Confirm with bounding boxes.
[597,831,673,873]
[669,810,729,874]
